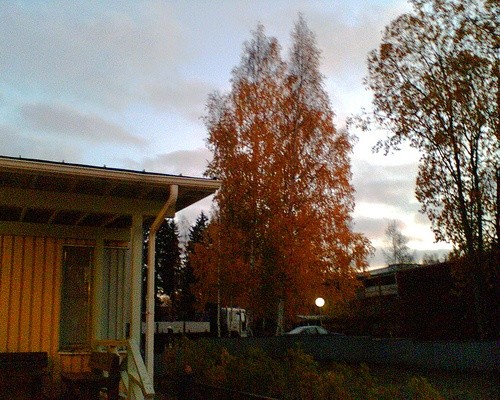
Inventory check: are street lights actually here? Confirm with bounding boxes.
[314,297,325,327]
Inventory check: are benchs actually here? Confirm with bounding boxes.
[0,351,49,395]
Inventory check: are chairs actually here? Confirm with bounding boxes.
[63,351,120,399]
